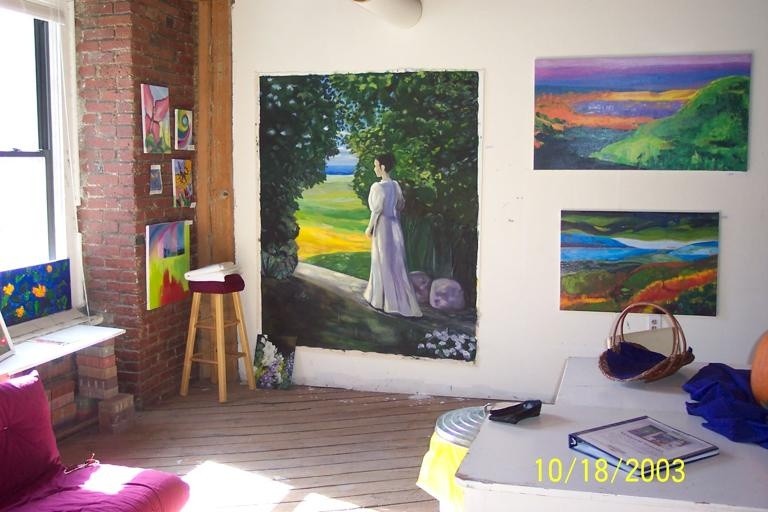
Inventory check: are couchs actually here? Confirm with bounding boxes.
[0,370,189,512]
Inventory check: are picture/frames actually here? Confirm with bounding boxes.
[0,311,15,361]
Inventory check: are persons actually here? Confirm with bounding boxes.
[362,154,423,317]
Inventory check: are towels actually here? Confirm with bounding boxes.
[185,261,241,282]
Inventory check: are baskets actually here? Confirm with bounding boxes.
[598,301,695,383]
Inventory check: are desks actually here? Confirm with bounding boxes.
[0,325,127,377]
[456,357,768,512]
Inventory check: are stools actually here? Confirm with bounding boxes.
[180,275,256,404]
